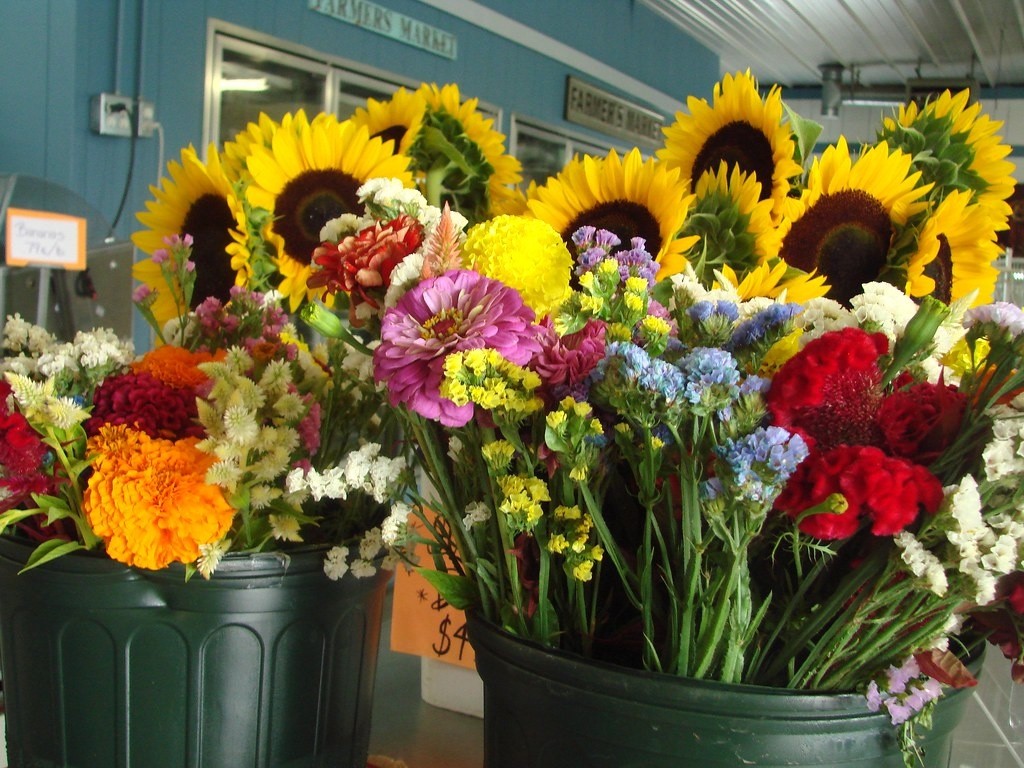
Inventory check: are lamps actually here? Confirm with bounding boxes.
[817,63,843,116]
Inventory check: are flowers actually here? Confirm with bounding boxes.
[0,67,1024,768]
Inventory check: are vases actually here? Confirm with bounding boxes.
[465,607,988,768]
[0,536,388,766]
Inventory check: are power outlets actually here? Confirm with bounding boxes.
[91,91,156,139]
